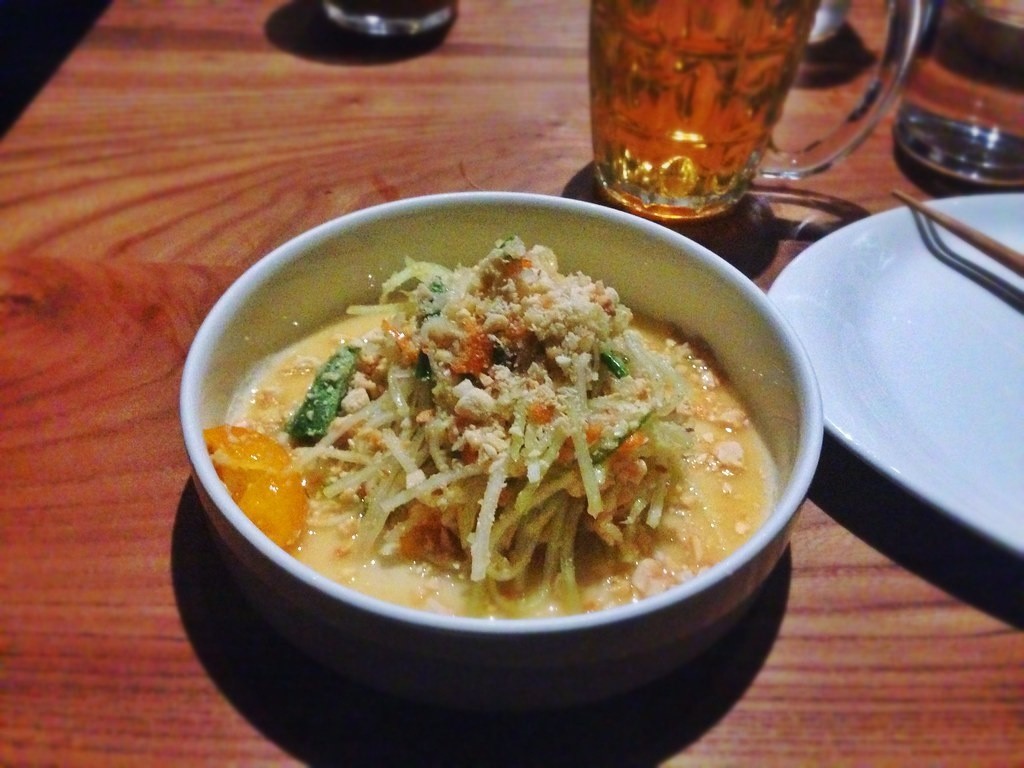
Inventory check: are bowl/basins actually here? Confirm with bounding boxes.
[181,189,824,667]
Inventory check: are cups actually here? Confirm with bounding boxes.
[891,0,1024,198]
[325,0,456,37]
[588,0,930,221]
[807,0,852,44]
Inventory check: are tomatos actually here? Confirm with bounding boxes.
[201,423,306,547]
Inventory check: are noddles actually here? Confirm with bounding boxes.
[238,287,765,623]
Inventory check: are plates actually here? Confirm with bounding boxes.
[767,193,1024,552]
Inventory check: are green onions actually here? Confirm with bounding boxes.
[288,235,659,469]
